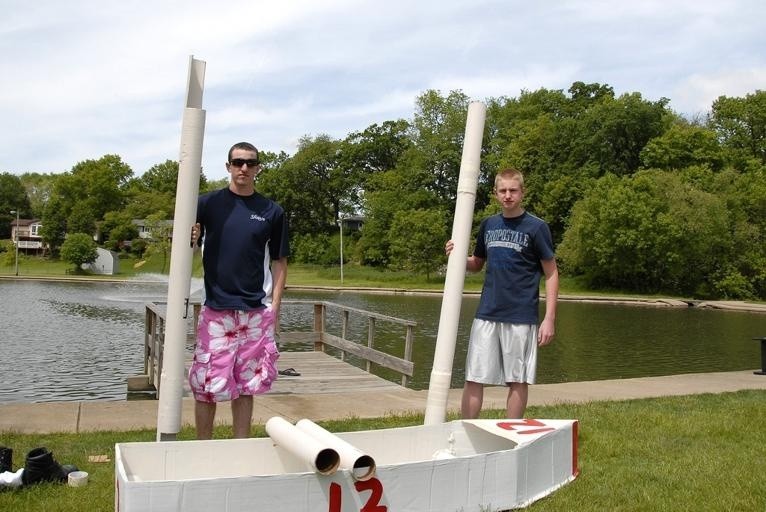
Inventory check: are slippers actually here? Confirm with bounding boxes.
[278,367,301,376]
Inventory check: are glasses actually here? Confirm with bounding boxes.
[229,157,259,167]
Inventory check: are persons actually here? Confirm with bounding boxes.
[192,142,291,440]
[445,170,559,419]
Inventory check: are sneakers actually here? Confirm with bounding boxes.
[21,446,79,484]
[0,446,14,490]
